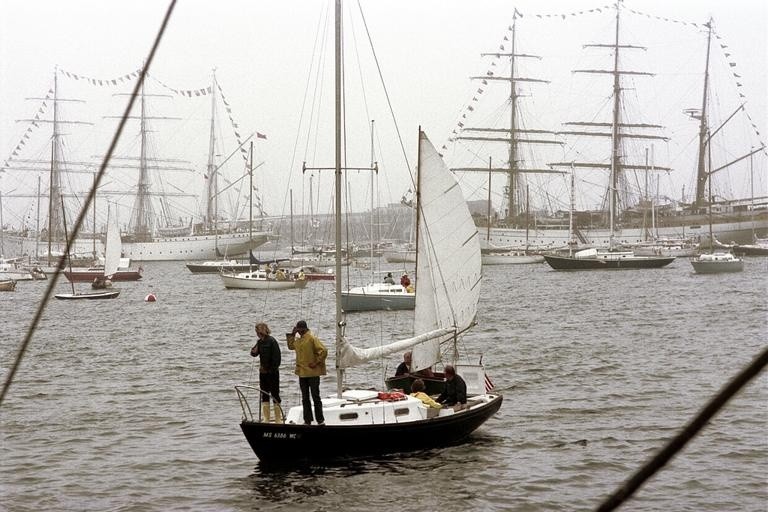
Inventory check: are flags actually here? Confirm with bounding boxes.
[537,4,709,29]
[213,78,265,219]
[60,69,213,98]
[712,29,767,157]
[256,132,267,140]
[0,76,58,179]
[437,14,518,160]
[479,363,493,394]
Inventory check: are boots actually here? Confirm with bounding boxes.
[274,403,281,424]
[262,402,270,423]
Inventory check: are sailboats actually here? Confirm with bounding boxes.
[2,57,284,262]
[186,141,408,290]
[480,146,768,270]
[234,1,503,470]
[400,1,768,256]
[689,132,747,273]
[333,120,417,313]
[0,170,145,291]
[53,195,123,300]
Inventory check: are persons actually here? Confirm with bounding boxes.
[285,320,327,426]
[250,323,281,425]
[395,352,411,376]
[408,378,443,410]
[266,269,305,281]
[382,271,414,294]
[434,366,466,406]
